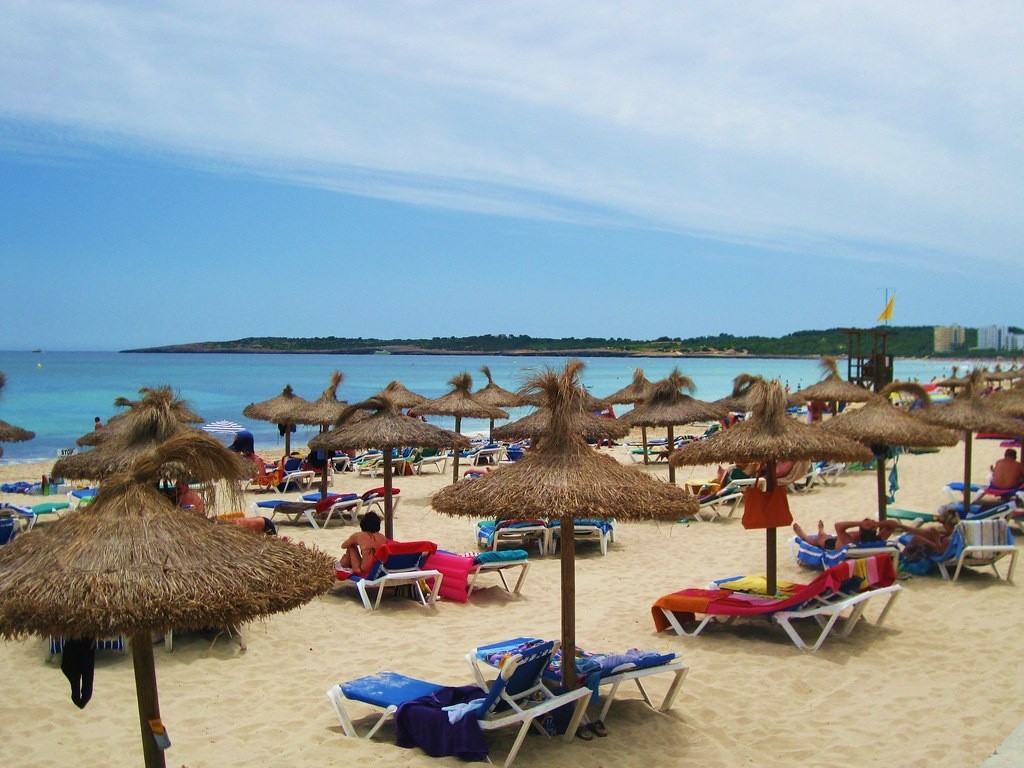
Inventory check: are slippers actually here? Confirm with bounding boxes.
[587,719,608,737]
[576,725,593,740]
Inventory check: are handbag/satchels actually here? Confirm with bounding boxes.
[742,458,794,529]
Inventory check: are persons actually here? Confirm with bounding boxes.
[775,459,796,477]
[95,417,103,430]
[340,510,388,579]
[175,480,208,519]
[235,516,277,535]
[793,517,898,550]
[595,405,616,449]
[731,459,761,479]
[463,466,496,479]
[897,507,960,541]
[990,448,1024,489]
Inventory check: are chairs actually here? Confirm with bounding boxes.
[326,639,593,767]
[653,552,905,655]
[787,480,1024,584]
[457,437,532,478]
[163,446,454,530]
[465,636,690,725]
[545,514,617,556]
[683,459,852,524]
[336,540,443,611]
[0,474,99,544]
[420,546,532,599]
[625,425,721,465]
[473,516,551,556]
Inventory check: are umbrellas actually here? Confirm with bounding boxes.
[0,360,1023,768]
